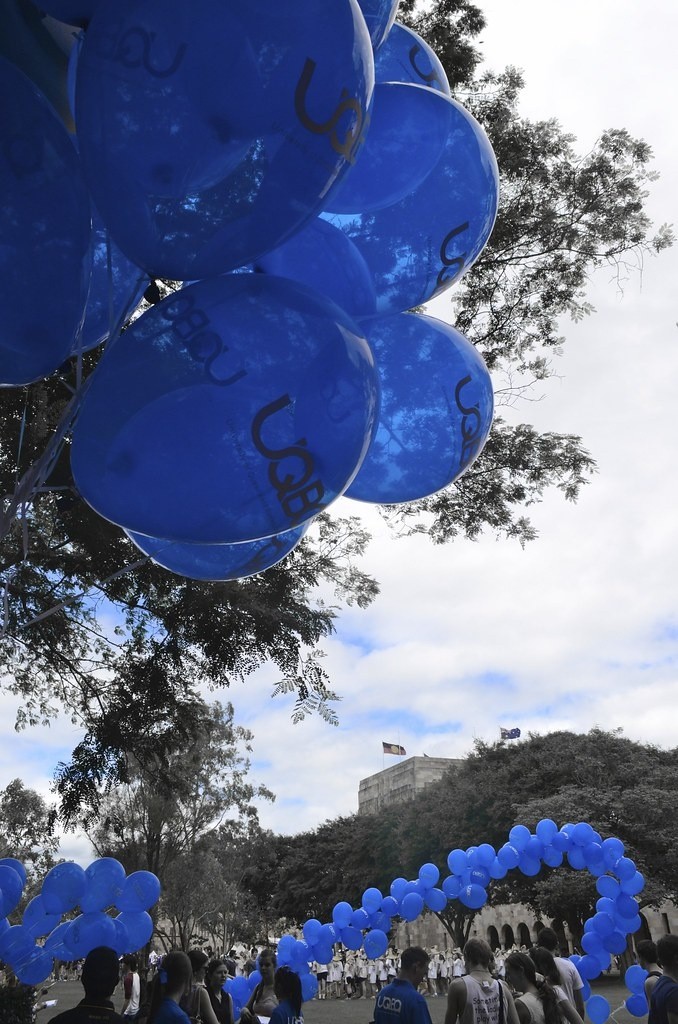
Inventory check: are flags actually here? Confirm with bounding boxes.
[501,729,520,739]
[382,743,407,755]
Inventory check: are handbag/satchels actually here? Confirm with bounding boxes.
[189,984,206,1024]
[239,982,263,1024]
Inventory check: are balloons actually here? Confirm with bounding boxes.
[207,814,650,1021]
[0,854,162,985]
[0,0,500,586]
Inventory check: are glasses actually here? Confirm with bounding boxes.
[201,965,210,971]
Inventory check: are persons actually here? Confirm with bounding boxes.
[0,935,677,1023]
